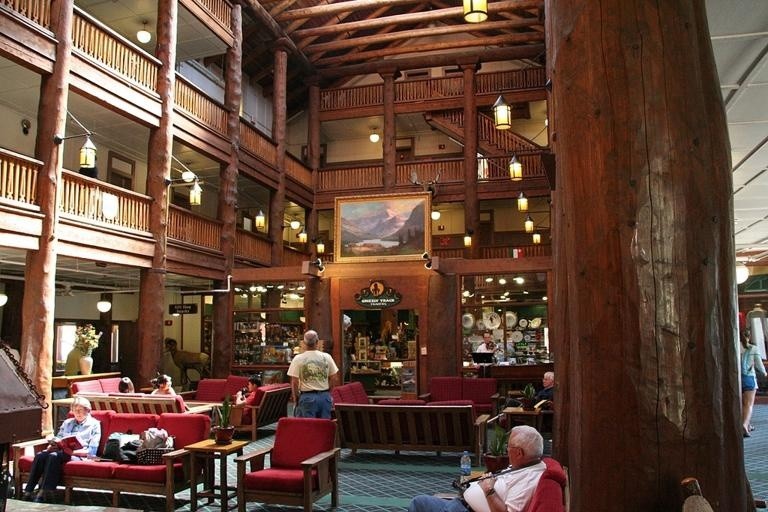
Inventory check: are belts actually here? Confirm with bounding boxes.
[301,390,329,394]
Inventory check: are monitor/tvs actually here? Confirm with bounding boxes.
[471,352,496,363]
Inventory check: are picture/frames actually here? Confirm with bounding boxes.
[331,189,432,264]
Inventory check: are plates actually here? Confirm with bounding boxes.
[463,312,542,341]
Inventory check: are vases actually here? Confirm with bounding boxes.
[77,356,94,375]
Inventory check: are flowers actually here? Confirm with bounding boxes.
[72,322,104,356]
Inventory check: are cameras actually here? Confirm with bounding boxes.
[452,480,471,499]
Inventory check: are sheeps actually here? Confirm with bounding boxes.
[162,337,211,385]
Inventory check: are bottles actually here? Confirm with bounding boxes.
[234,321,298,365]
[459,449,472,486]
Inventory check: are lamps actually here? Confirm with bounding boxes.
[232,203,267,232]
[430,209,440,222]
[281,223,308,243]
[503,121,547,183]
[487,46,544,129]
[529,224,551,246]
[366,125,381,143]
[311,234,325,254]
[50,132,98,170]
[522,212,535,235]
[93,289,116,314]
[135,19,152,44]
[288,211,302,231]
[460,0,489,26]
[163,172,205,205]
[516,189,530,210]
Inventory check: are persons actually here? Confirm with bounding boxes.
[505,371,554,409]
[287,330,339,419]
[20,396,103,503]
[119,377,134,392]
[471,331,499,370]
[738,328,767,436]
[408,424,547,512]
[150,374,190,412]
[235,376,262,418]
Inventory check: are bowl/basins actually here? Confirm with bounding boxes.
[468,352,493,363]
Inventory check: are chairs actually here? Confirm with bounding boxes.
[671,478,720,512]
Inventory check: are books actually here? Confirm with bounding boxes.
[48,434,83,453]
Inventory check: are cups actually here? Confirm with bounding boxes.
[90,445,98,456]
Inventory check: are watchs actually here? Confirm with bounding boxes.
[485,487,496,497]
